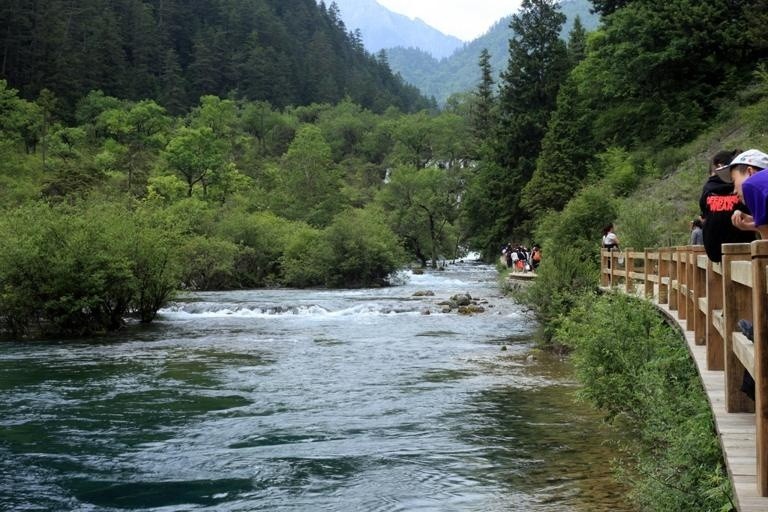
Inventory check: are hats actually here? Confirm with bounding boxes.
[712,149,738,166]
[714,148,768,184]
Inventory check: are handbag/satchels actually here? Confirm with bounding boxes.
[524,263,531,270]
[517,259,525,269]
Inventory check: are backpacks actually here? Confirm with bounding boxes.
[532,250,541,261]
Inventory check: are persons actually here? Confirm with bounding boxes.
[495,242,544,273]
[696,214,706,223]
[700,150,756,261]
[601,223,619,284]
[714,149,768,347]
[688,219,703,245]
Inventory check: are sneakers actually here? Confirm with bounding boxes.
[736,316,755,342]
